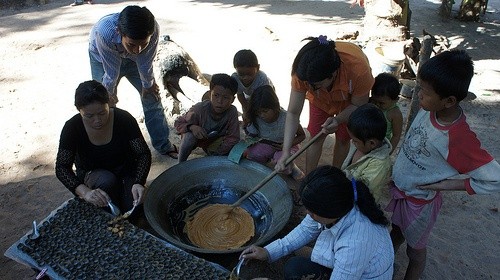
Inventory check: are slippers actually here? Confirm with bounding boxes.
[165,144,179,159]
[291,166,305,180]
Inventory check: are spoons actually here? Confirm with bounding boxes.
[28,219,40,240]
[230,258,244,280]
[107,200,120,216]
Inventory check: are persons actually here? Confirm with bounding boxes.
[175,74,239,163]
[239,165,393,280]
[372,73,404,147]
[387,50,500,280]
[275,36,374,204]
[246,84,306,183]
[154,35,210,111]
[89,5,178,160]
[340,104,392,207]
[232,49,276,123]
[56,80,152,224]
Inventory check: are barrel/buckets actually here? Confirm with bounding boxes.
[366,42,405,81]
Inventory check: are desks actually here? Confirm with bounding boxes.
[4,197,230,280]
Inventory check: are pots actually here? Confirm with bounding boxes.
[143,135,293,253]
